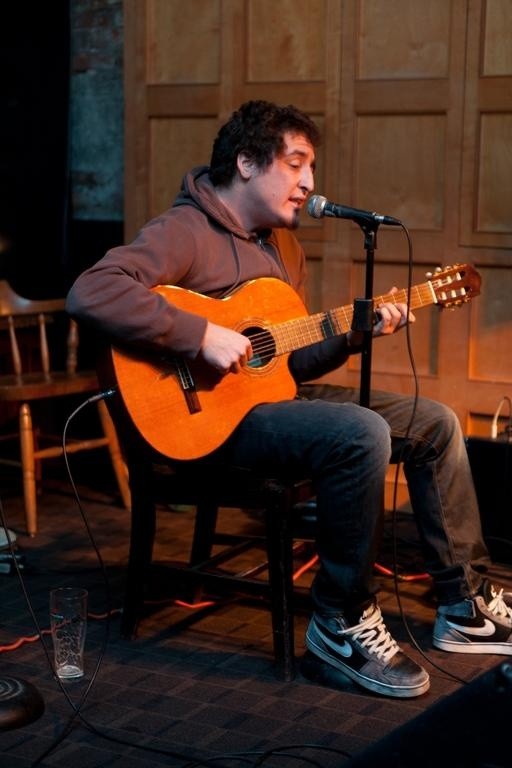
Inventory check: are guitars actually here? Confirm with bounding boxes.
[111,264,482,460]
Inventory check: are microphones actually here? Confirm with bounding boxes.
[306,194,401,226]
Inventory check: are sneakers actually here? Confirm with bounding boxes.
[431,579,512,656]
[306,594,430,698]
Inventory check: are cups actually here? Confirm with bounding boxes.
[47,585,88,683]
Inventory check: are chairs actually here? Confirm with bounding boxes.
[99,354,320,681]
[0,282,130,537]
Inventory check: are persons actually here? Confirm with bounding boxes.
[63,97,512,699]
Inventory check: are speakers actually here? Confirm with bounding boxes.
[464,426,512,570]
